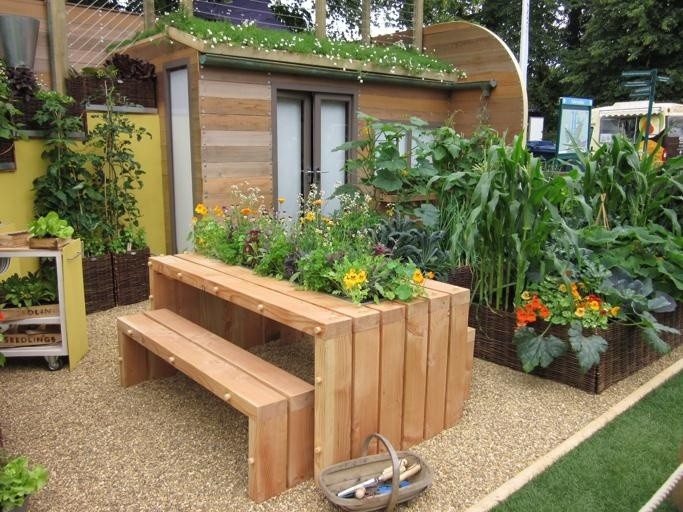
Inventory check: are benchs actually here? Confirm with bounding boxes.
[116,308,315,503]
[463,327,475,400]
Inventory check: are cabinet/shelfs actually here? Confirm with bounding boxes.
[0,239,84,371]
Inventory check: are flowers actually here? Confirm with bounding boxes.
[184,181,434,306]
[514,272,623,333]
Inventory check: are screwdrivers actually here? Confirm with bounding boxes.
[337,458,422,500]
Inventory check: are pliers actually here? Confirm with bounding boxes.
[343,479,411,498]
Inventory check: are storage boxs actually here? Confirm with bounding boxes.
[0,333,61,347]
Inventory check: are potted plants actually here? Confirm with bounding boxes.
[102,51,156,107]
[26,211,74,248]
[30,89,115,315]
[0,267,59,321]
[81,65,149,306]
[4,67,45,130]
[65,66,109,105]
[325,109,682,394]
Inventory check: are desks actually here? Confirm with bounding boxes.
[147,251,471,487]
